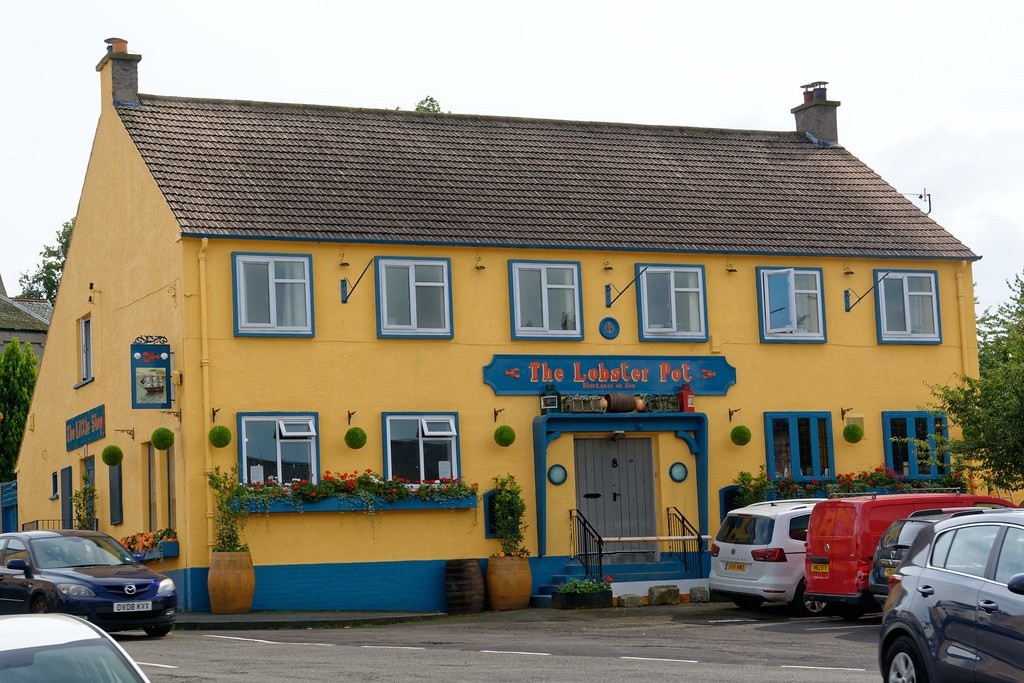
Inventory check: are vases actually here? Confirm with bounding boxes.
[231,491,478,513]
[574,589,612,608]
[209,543,255,614]
[551,591,577,608]
[749,483,969,500]
[487,553,535,610]
[128,540,179,561]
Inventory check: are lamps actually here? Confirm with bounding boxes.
[338,250,352,266]
[727,263,738,273]
[610,430,625,443]
[475,257,485,270]
[843,264,855,275]
[603,258,614,270]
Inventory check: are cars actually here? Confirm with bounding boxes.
[0,612,150,683]
[869,506,999,610]
[706,498,831,615]
[878,509,1023,683]
[0,531,178,638]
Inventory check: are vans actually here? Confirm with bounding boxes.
[804,488,1024,618]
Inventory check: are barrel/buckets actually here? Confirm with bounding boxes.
[444,558,485,615]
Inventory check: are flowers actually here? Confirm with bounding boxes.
[732,463,972,499]
[120,527,179,550]
[555,575,614,592]
[205,465,260,550]
[237,469,478,505]
[489,471,534,559]
